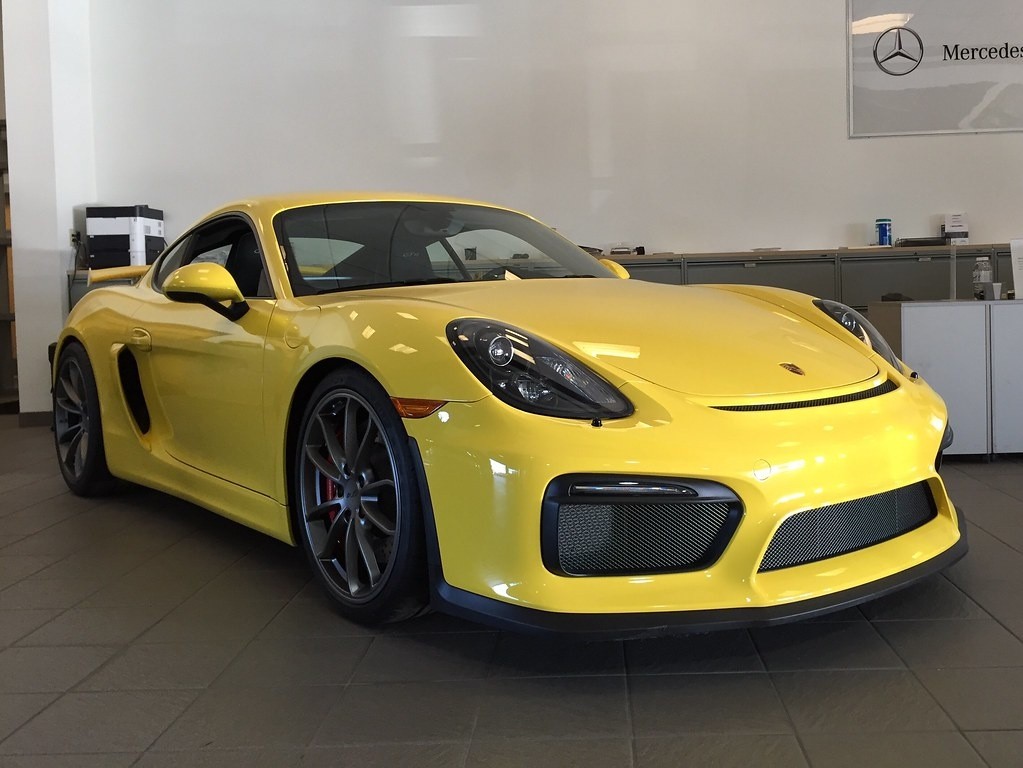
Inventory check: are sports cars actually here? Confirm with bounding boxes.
[48,193,972,642]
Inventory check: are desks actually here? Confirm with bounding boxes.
[430,237,1023,465]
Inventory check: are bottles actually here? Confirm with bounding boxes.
[874,218,893,248]
[972,256,994,300]
